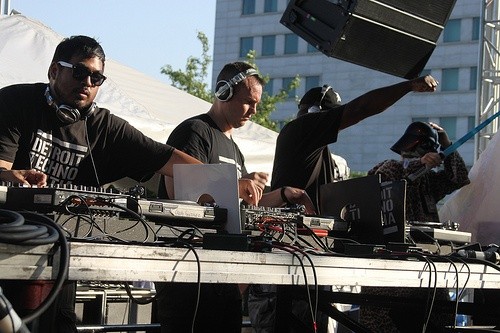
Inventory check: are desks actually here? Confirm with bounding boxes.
[0,242,500,333]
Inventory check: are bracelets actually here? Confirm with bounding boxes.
[281,186,288,202]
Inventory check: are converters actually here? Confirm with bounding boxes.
[344,241,416,260]
[202,233,248,251]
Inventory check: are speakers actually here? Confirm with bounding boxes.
[280,0,457,81]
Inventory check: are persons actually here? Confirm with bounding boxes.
[157,61,316,333]
[248,74,440,333]
[360,121,470,333]
[0,36,262,333]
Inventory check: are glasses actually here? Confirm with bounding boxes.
[57,61,107,86]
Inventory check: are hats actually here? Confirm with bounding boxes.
[297,86,342,109]
[389,121,439,158]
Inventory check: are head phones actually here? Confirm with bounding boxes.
[44,85,97,125]
[308,84,333,113]
[215,68,259,101]
